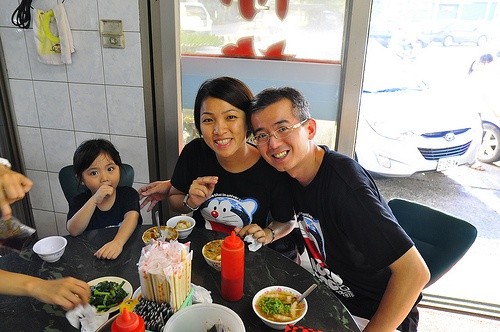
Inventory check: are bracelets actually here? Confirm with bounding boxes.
[266,226,275,243]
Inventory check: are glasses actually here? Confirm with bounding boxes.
[252,118,307,145]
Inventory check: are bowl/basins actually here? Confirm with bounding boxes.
[252,285,308,330]
[142,226,179,245]
[202,240,224,271]
[32,236,67,263]
[166,216,196,239]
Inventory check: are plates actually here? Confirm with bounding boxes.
[75,276,133,315]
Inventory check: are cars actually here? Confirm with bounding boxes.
[181,3,214,33]
[250,9,499,181]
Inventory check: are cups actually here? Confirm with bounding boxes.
[163,303,246,332]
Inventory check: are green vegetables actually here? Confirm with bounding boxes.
[258,296,291,315]
[87,280,129,312]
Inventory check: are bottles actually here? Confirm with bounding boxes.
[220,231,245,301]
[110,308,146,332]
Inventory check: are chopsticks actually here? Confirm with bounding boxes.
[136,240,193,311]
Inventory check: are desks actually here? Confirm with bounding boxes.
[0,222,363,332]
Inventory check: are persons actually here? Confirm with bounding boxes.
[168,76,306,266]
[0,158,91,309]
[139,87,430,332]
[66,139,143,259]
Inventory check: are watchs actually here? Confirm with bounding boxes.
[183,194,199,212]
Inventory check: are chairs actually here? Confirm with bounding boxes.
[59,163,134,203]
[388,197,478,290]
